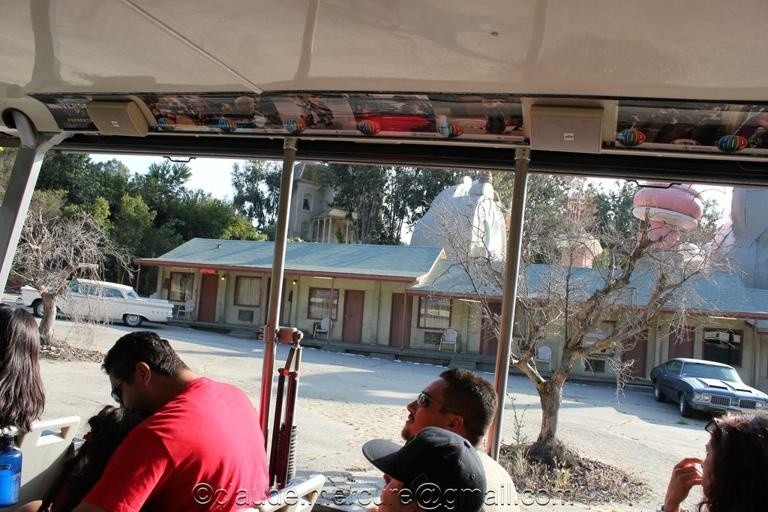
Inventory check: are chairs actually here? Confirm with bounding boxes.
[534,345,552,373]
[176,300,196,321]
[439,328,458,353]
[0,414,81,512]
[236,473,325,512]
[313,317,333,341]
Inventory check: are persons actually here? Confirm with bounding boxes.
[0,305,272,512]
[658,409,768,512]
[366,368,520,512]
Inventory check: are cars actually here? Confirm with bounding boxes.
[650,357,768,417]
[16,278,174,327]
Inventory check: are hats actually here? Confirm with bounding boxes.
[363,426,486,512]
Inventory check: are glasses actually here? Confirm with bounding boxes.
[111,380,124,403]
[705,417,722,433]
[417,391,459,416]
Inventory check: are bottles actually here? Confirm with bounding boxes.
[0,435,22,506]
